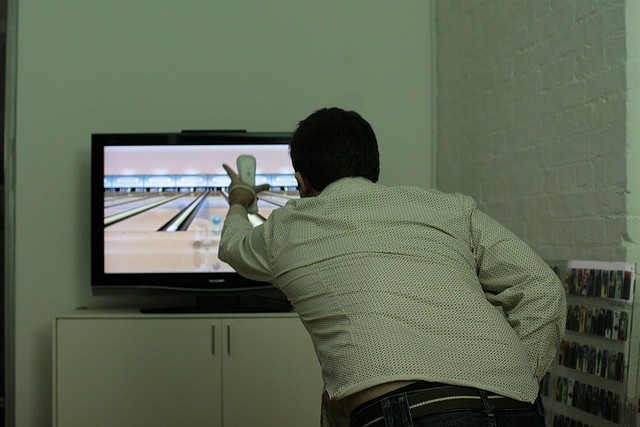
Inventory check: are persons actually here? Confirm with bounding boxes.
[218,108,567,427]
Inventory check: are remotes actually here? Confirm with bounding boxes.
[235,151,260,216]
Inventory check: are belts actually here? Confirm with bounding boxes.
[350,387,544,426]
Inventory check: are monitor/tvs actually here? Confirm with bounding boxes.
[89,128,295,311]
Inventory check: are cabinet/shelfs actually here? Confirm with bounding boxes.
[49,307,329,425]
